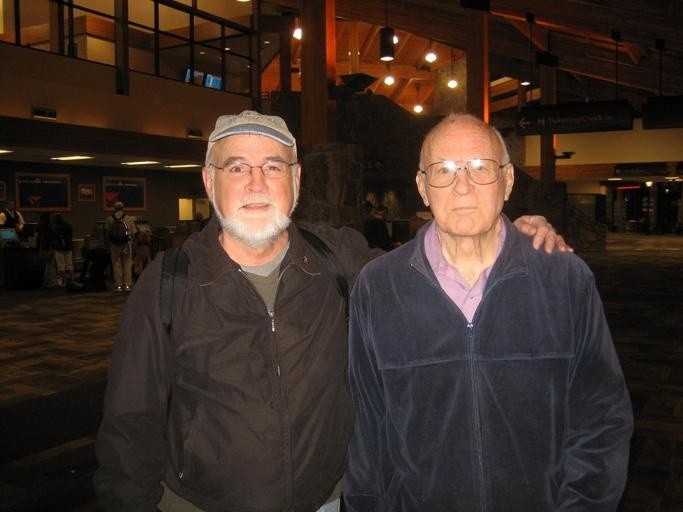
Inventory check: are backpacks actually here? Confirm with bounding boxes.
[110,213,129,243]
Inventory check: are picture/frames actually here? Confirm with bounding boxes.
[0,179,7,202]
[76,183,96,201]
[101,176,147,211]
[14,171,72,213]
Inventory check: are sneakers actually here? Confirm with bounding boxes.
[114,285,132,291]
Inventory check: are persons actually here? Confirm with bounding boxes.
[79,246,112,278]
[104,201,139,293]
[351,200,402,251]
[92,109,577,512]
[53,212,74,288]
[340,111,637,512]
[36,213,65,289]
[0,199,26,226]
[375,206,388,220]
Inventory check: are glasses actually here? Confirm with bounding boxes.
[209,163,295,178]
[422,159,503,187]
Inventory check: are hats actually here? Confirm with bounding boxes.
[209,110,296,147]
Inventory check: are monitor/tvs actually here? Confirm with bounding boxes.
[184,67,205,87]
[22,223,38,237]
[205,73,222,90]
[0,229,20,243]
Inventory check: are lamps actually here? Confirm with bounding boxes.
[380,1,395,62]
